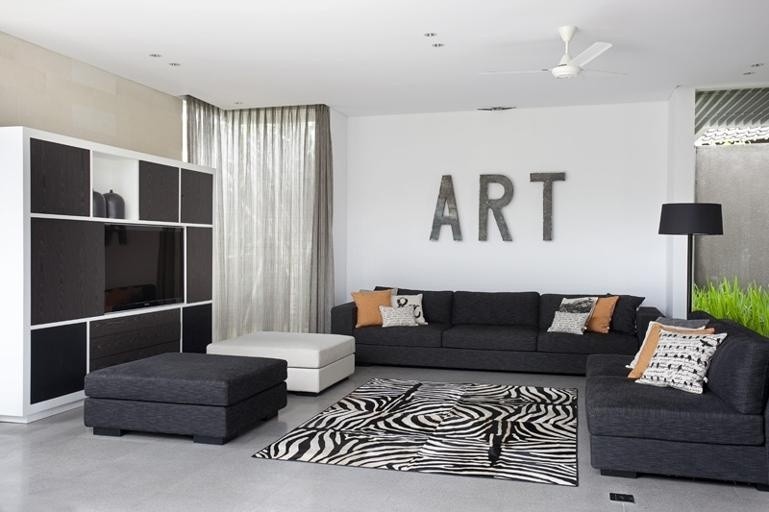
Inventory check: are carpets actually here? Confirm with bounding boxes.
[252,375,580,491]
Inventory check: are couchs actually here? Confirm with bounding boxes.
[583,310,768,492]
[80,348,292,445]
[204,331,358,398]
[328,286,665,379]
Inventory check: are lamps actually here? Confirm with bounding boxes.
[655,202,726,320]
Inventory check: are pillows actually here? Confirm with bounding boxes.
[626,324,716,386]
[391,291,429,325]
[655,316,710,329]
[546,310,587,334]
[378,304,420,329]
[350,289,396,329]
[625,320,707,370]
[558,295,600,319]
[632,326,728,395]
[605,293,646,335]
[586,297,620,335]
[364,288,399,304]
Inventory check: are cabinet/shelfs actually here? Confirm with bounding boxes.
[0,125,217,425]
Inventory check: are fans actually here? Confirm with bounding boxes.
[476,22,629,83]
